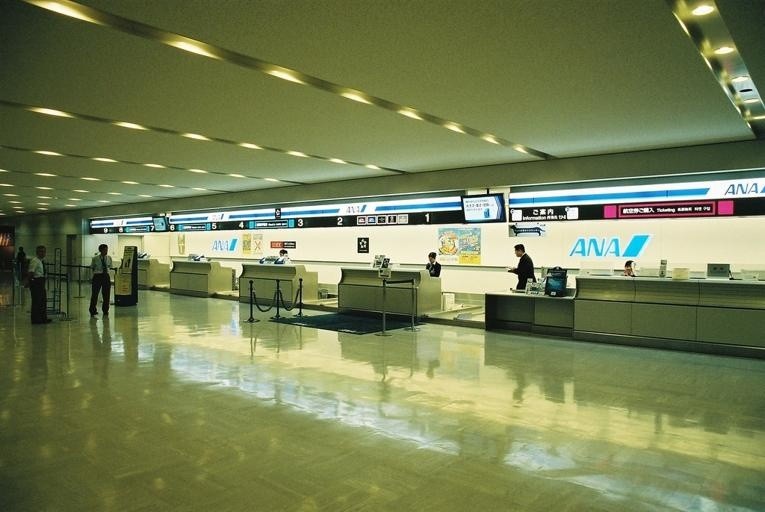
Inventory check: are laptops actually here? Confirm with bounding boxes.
[707,263,730,280]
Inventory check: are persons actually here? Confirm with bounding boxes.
[507,243,537,294]
[426,252,442,278]
[16,247,26,281]
[623,260,636,277]
[24,246,52,324]
[280,250,287,257]
[89,243,113,316]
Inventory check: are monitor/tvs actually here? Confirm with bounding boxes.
[461,193,507,224]
[545,276,567,297]
[152,216,168,232]
[672,267,689,280]
[547,269,567,277]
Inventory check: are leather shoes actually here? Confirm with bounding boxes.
[103,311,109,316]
[31,319,51,324]
[91,312,98,315]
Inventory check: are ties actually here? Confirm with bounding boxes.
[42,260,45,275]
